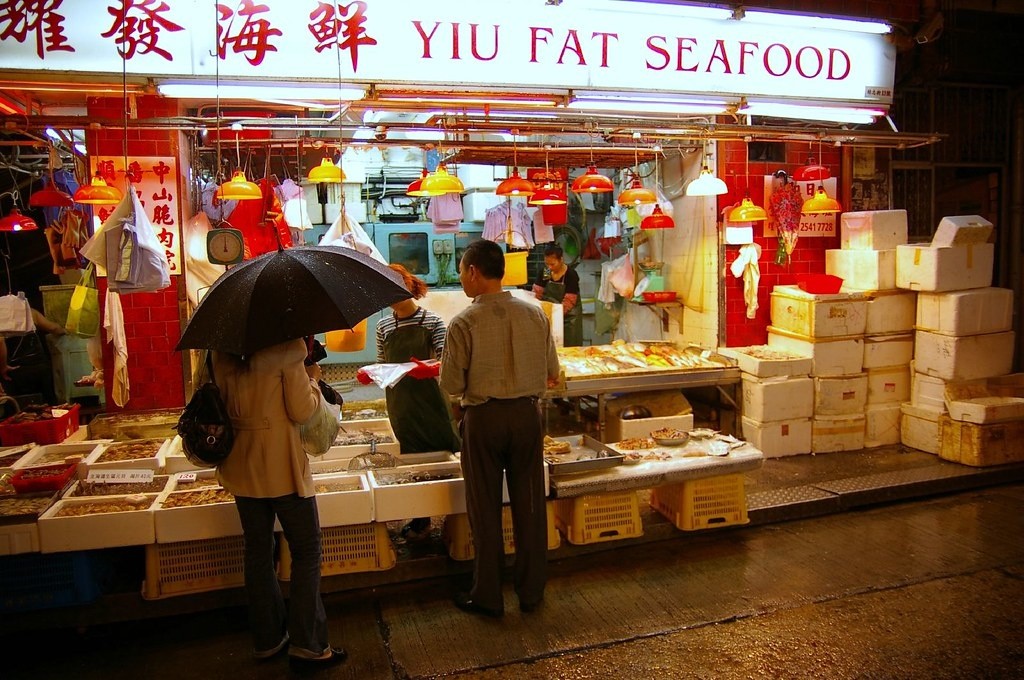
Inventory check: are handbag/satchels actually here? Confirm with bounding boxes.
[65,262,100,338]
[297,386,342,456]
[175,349,234,469]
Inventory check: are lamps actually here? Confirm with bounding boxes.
[686,127,727,198]
[801,133,841,213]
[405,117,676,230]
[216,123,263,199]
[728,135,767,222]
[0,123,123,232]
[307,146,346,183]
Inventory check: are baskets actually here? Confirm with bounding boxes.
[7,463,77,493]
[0,404,80,446]
[642,292,676,303]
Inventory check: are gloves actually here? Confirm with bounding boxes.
[356,372,373,385]
[407,357,440,379]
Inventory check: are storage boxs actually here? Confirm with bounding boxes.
[0,208,1024,601]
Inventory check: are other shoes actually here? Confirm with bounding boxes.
[519,587,543,612]
[288,648,347,675]
[253,630,290,663]
[453,593,504,618]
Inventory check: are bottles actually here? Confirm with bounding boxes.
[604,211,610,222]
[773,240,786,265]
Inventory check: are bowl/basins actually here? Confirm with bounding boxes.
[620,405,652,420]
[650,430,689,446]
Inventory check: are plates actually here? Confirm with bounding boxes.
[621,427,715,465]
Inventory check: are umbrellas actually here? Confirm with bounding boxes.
[172,240,416,357]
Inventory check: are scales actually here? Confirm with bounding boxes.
[207,213,245,272]
[630,262,664,302]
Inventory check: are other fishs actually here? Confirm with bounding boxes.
[615,426,747,466]
[557,338,734,377]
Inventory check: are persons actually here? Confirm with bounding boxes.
[0,256,69,417]
[437,238,566,625]
[193,334,349,668]
[355,263,460,454]
[532,240,584,346]
